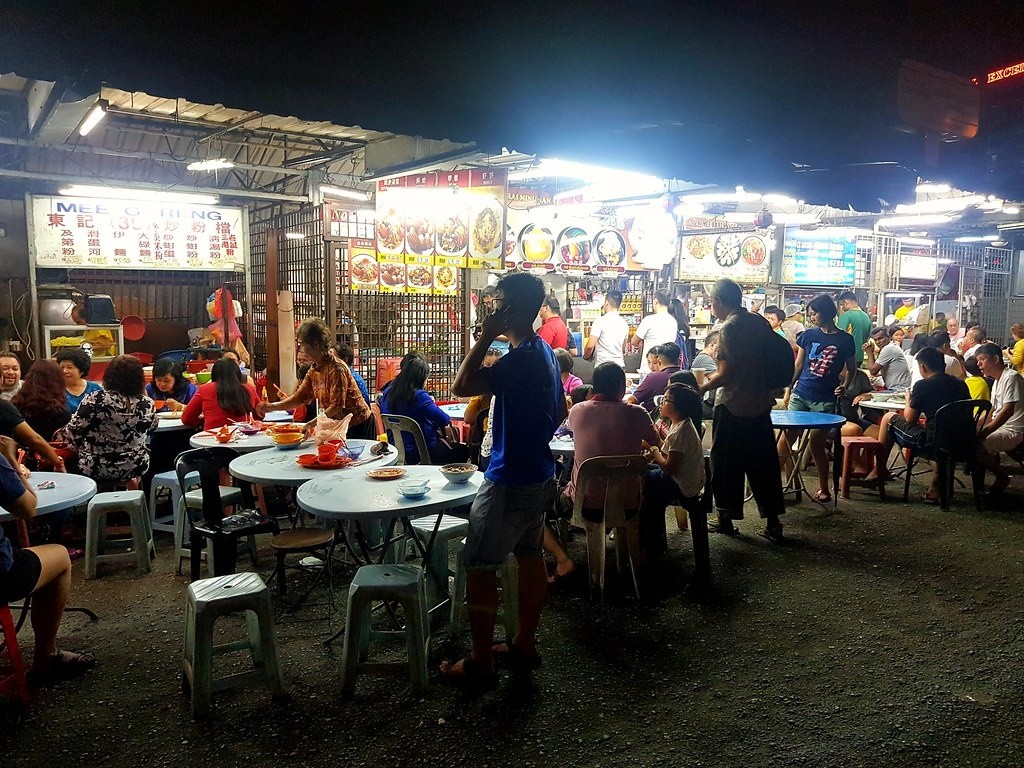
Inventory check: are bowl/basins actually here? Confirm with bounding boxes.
[337,440,366,460]
[439,463,479,484]
[11,344,23,352]
[297,440,342,467]
[129,352,154,365]
[160,350,195,374]
[871,393,892,401]
[186,360,216,374]
[270,424,305,449]
[153,400,166,410]
[196,372,212,384]
[215,421,263,443]
[395,478,430,498]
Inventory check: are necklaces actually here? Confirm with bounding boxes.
[70,390,77,395]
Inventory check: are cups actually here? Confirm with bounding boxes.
[691,367,705,385]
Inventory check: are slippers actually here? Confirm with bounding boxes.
[439,654,496,681]
[492,638,537,660]
[548,570,577,586]
[812,490,831,502]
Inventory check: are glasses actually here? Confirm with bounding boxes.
[662,397,674,405]
[491,299,504,308]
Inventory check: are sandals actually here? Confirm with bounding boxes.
[31,648,96,677]
[707,521,739,533]
[756,525,783,539]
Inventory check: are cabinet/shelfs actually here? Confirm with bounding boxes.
[44,324,125,385]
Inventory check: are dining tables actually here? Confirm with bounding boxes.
[853,386,936,494]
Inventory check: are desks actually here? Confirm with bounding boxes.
[224,436,400,610]
[295,463,488,647]
[188,422,316,450]
[764,408,847,515]
[0,470,99,547]
[149,408,202,434]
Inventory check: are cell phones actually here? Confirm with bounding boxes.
[499,301,517,314]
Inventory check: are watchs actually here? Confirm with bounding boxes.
[54,455,64,467]
[650,446,658,453]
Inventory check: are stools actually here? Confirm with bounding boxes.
[397,512,450,629]
[173,483,264,577]
[149,471,202,545]
[338,560,436,702]
[176,569,287,719]
[448,538,523,651]
[269,526,337,629]
[840,435,891,500]
[81,489,158,583]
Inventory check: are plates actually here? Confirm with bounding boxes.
[351,199,626,292]
[306,456,351,467]
[120,314,146,341]
[713,232,769,268]
[260,424,273,430]
[365,468,408,480]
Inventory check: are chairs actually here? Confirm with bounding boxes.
[381,413,431,465]
[554,453,653,602]
[664,456,731,574]
[170,442,288,599]
[0,603,30,704]
[901,397,996,513]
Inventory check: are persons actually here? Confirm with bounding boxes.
[144,358,199,505]
[435,275,569,688]
[55,347,102,414]
[10,359,74,472]
[631,288,678,385]
[0,350,26,402]
[255,284,1024,598]
[583,290,629,384]
[537,295,568,350]
[776,295,857,504]
[0,434,96,676]
[181,357,265,520]
[0,388,87,558]
[204,348,256,392]
[319,298,358,349]
[52,354,158,518]
[697,278,787,544]
